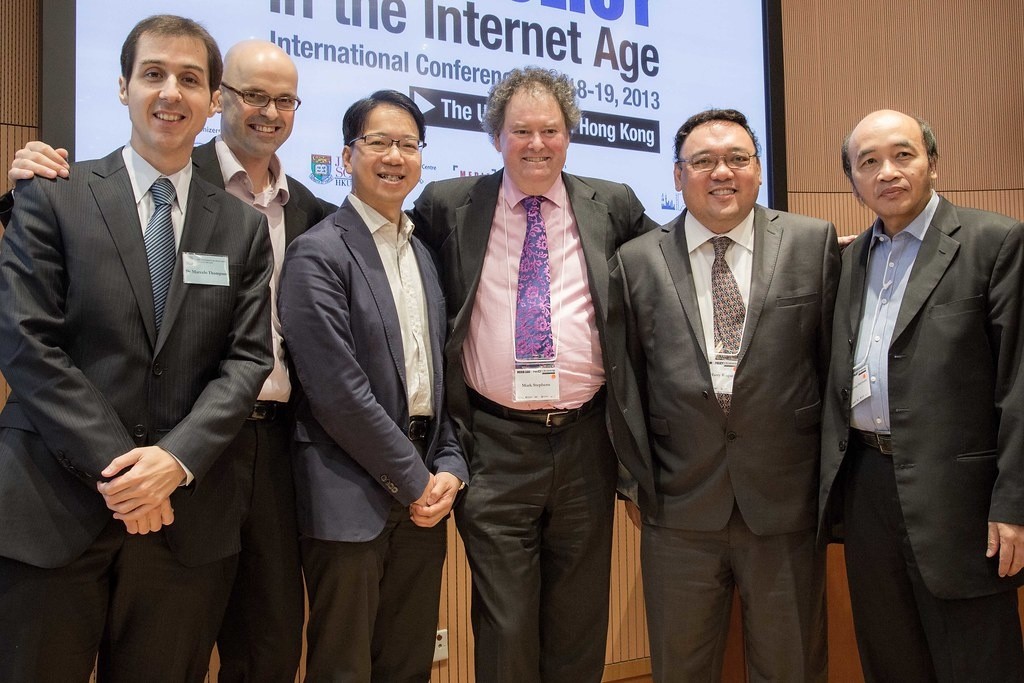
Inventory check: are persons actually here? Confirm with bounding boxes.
[402,66,859,683]
[279,90,469,683]
[0,14,275,683]
[816,109,1024,683]
[0,39,339,683]
[618,109,842,683]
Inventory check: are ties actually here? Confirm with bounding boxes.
[708,235,746,417]
[514,195,555,369]
[143,177,174,335]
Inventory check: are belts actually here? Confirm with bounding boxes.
[468,392,590,428]
[406,417,430,441]
[246,401,289,425]
[851,430,892,455]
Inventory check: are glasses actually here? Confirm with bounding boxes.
[348,133,427,156]
[676,152,758,171]
[220,81,301,111]
